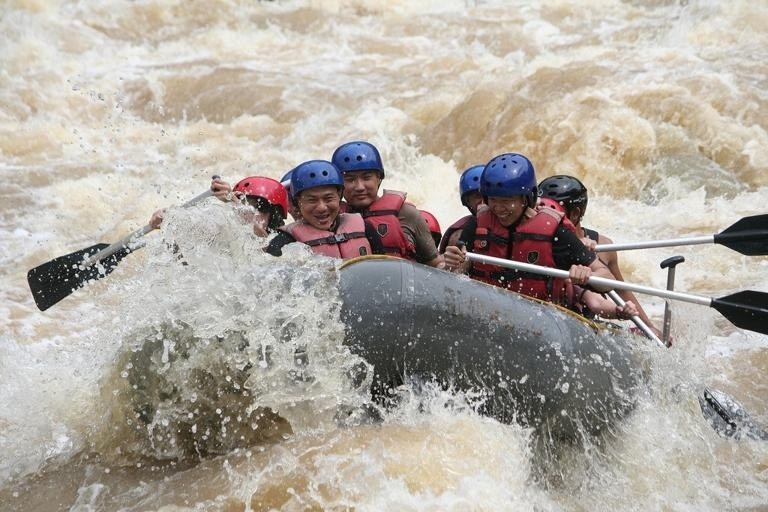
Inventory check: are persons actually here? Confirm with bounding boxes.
[440,153,673,348]
[149,141,441,269]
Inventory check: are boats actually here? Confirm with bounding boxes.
[119,253,655,447]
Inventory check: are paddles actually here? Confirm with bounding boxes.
[589,214,768,256]
[462,249,768,335]
[607,286,768,442]
[28,174,223,311]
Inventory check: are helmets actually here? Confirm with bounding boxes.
[460,165,486,207]
[232,176,288,219]
[281,168,295,199]
[479,154,535,196]
[537,175,588,215]
[289,159,344,200]
[332,141,385,180]
